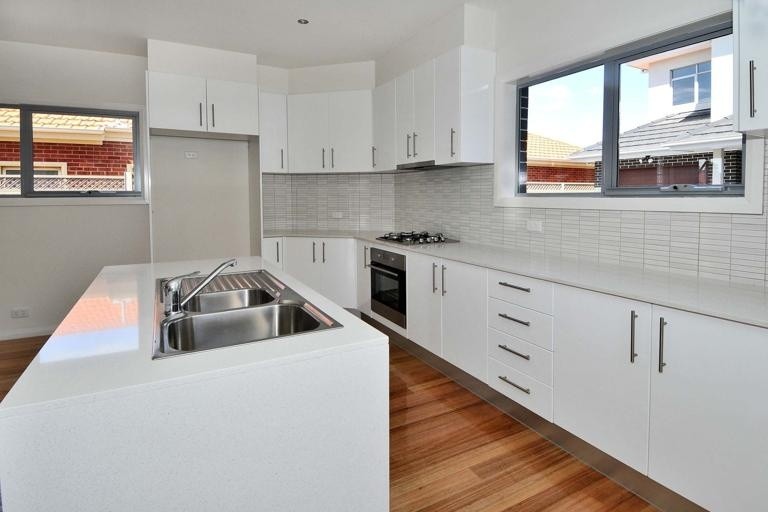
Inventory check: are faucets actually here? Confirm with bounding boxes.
[163,257,238,315]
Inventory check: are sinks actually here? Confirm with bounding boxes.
[151,301,343,361]
[151,267,279,314]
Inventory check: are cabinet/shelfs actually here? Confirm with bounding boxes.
[435,59,494,168]
[407,252,487,387]
[148,42,260,149]
[282,236,357,311]
[290,66,372,176]
[258,66,288,173]
[264,237,282,272]
[732,0,763,130]
[396,63,434,164]
[552,284,768,512]
[483,268,553,427]
[373,80,397,175]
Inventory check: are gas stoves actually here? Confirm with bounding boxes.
[376,230,461,246]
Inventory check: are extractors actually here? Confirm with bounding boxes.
[398,161,461,173]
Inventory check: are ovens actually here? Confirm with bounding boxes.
[367,262,406,329]
